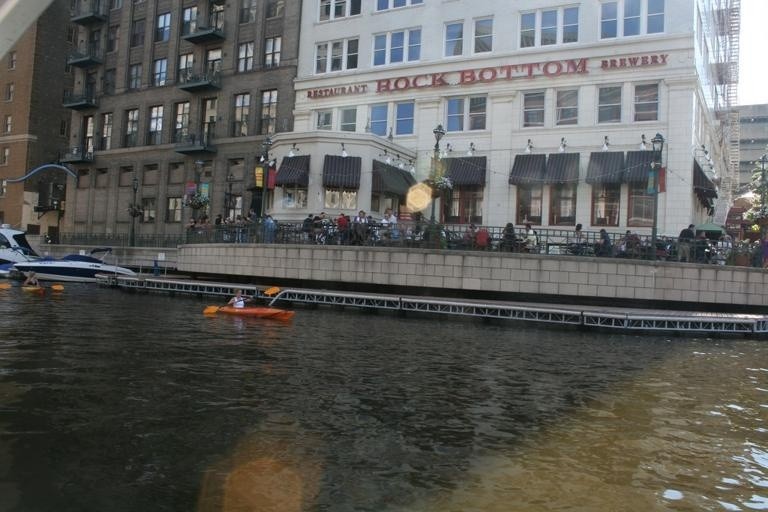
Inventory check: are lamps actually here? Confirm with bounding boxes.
[258,141,418,175]
[695,142,716,176]
[440,134,651,158]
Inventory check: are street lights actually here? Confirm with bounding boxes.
[57,184,64,243]
[431,124,446,222]
[652,133,664,234]
[226,174,234,217]
[196,160,204,194]
[261,136,272,217]
[758,155,768,240]
[131,177,139,247]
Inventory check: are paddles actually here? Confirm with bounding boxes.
[0,283,64,291]
[203,287,279,314]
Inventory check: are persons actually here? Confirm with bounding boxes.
[227,288,252,308]
[598,229,610,256]
[571,224,588,254]
[184,209,277,244]
[463,224,490,251]
[303,209,400,246]
[500,223,538,253]
[619,224,768,267]
[24,272,39,286]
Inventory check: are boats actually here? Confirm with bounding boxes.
[19,285,47,295]
[219,306,296,320]
[0,223,139,283]
